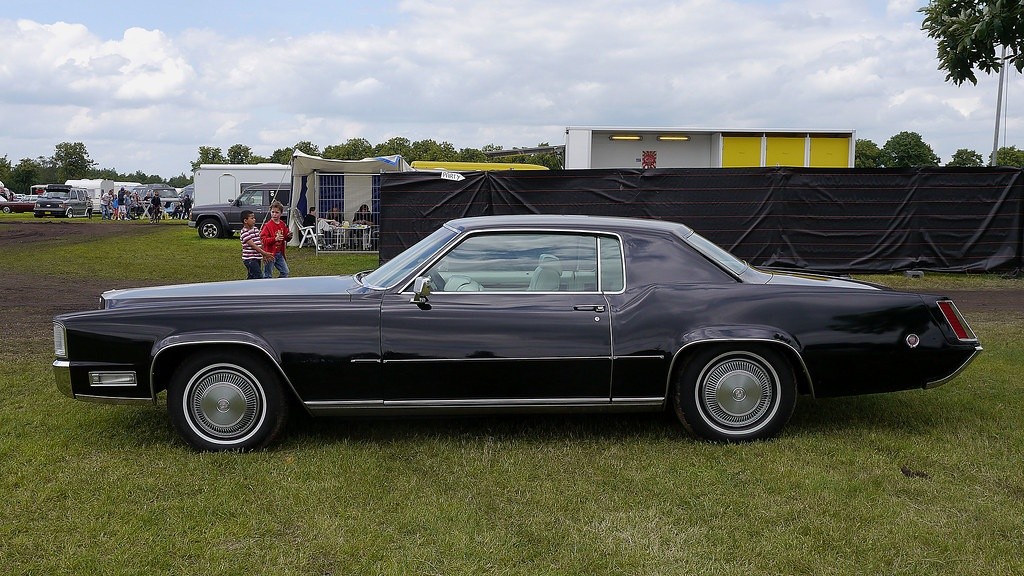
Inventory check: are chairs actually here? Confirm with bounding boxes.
[442,276,485,292]
[529,254,562,291]
[292,208,326,250]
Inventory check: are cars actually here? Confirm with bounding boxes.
[52,213,984,456]
[0,194,41,214]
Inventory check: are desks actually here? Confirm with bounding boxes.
[136,201,151,220]
[330,224,371,250]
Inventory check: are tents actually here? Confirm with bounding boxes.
[290,147,404,255]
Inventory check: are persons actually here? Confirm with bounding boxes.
[85,186,193,223]
[303,204,375,250]
[261,201,294,278]
[239,210,276,279]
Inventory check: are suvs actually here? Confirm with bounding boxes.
[187,182,292,239]
[180,181,195,211]
[33,184,94,219]
[130,183,182,220]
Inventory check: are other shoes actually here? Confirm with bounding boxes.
[118,218,120,221]
[326,244,334,249]
[124,218,130,221]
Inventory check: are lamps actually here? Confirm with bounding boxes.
[656,136,692,141]
[608,136,643,140]
